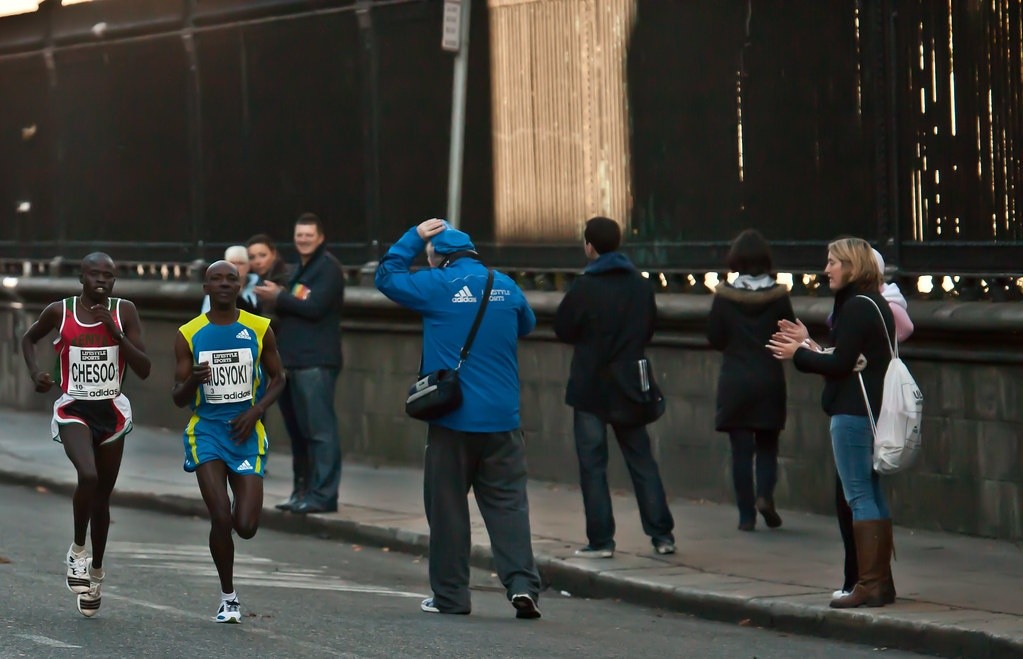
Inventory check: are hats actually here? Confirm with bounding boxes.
[429,218,476,255]
[871,248,885,276]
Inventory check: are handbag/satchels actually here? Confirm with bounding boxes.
[633,357,666,424]
[405,367,463,421]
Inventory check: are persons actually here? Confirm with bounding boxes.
[555,216,677,558]
[174,261,286,624]
[248,235,305,510]
[705,230,797,530]
[21,252,151,616]
[375,219,542,618]
[253,214,344,514]
[766,238,914,609]
[201,246,258,313]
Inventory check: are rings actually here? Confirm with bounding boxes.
[780,352,782,355]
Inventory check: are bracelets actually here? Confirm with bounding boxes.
[112,333,125,342]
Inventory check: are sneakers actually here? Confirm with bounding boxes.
[421,597,441,611]
[216,595,241,622]
[511,594,541,619]
[77,558,106,617]
[651,533,675,553]
[577,540,616,556]
[65,541,92,594]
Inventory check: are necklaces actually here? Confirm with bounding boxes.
[80,295,93,312]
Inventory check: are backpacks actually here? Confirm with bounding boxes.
[854,293,923,475]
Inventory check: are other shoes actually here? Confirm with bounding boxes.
[737,504,757,530]
[756,496,782,527]
[832,589,850,599]
[293,500,337,514]
[276,489,306,510]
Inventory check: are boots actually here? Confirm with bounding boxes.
[829,518,897,608]
